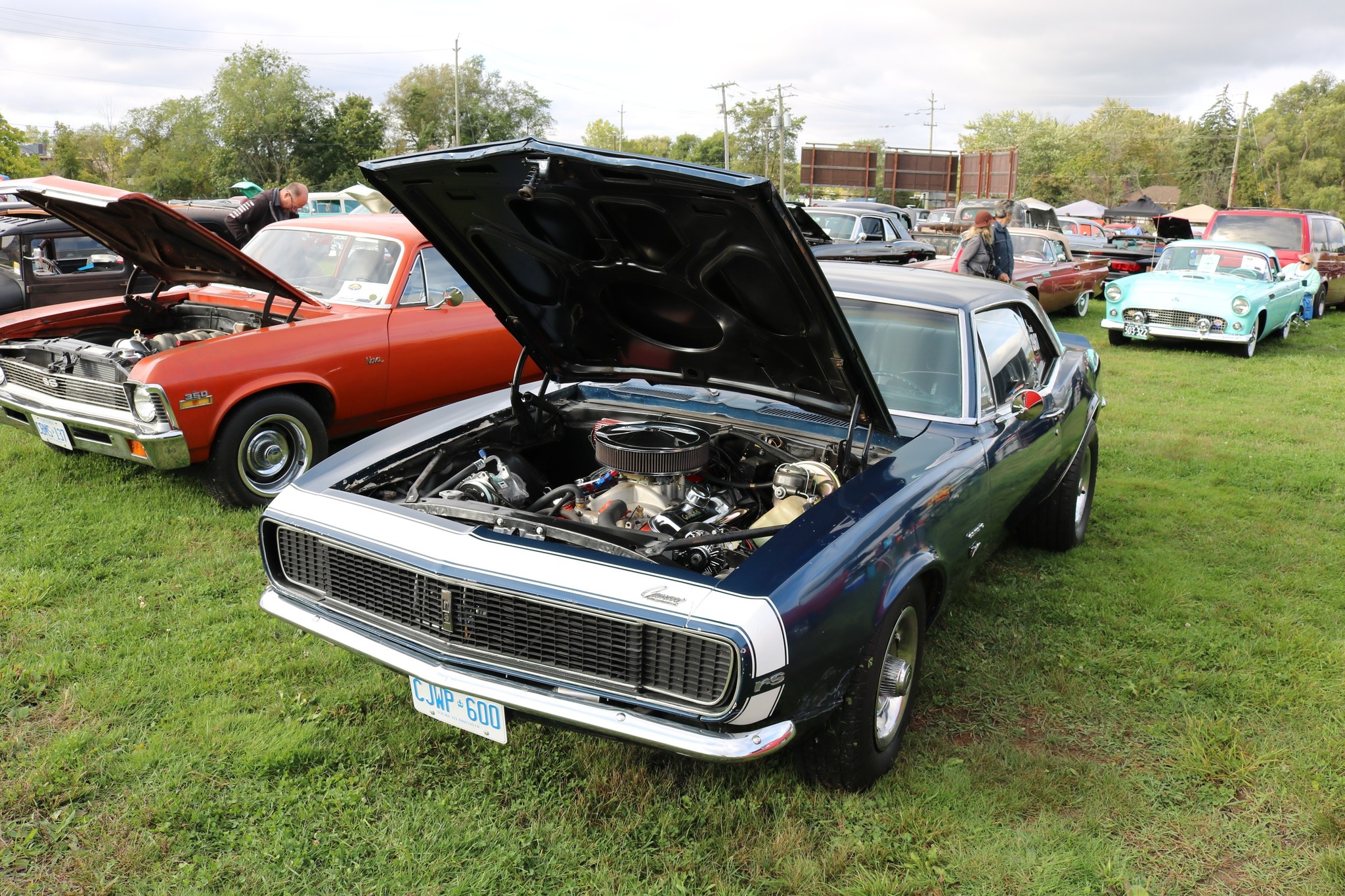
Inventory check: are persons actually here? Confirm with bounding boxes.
[1124,220,1143,244]
[224,181,308,249]
[957,210,997,278]
[1064,212,1070,216]
[988,198,1014,284]
[1281,253,1321,296]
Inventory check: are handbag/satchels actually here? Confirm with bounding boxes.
[951,235,981,272]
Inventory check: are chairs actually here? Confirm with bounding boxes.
[334,249,384,288]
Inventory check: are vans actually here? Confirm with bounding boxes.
[1195,207,1345,320]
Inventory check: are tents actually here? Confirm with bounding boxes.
[1019,196,1109,219]
[1102,194,1173,224]
[1163,203,1219,223]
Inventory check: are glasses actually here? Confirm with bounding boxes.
[1300,261,1311,265]
[287,191,302,210]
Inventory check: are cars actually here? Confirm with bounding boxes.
[0,180,407,258]
[784,197,1207,302]
[1101,240,1307,359]
[902,226,1111,318]
[256,135,1107,795]
[1,175,546,515]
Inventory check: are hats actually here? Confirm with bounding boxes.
[973,210,995,228]
[1131,221,1136,224]
